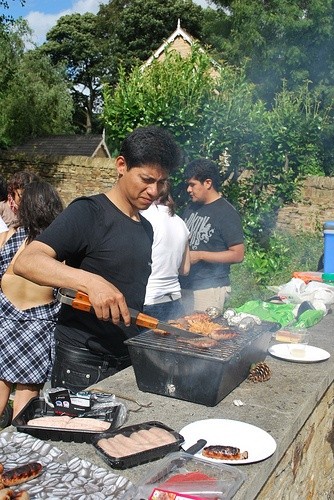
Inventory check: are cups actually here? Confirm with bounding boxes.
[291,332,310,358]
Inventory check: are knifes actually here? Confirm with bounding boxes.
[146,439,207,484]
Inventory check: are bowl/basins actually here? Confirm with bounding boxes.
[91,420,185,470]
[11,395,122,444]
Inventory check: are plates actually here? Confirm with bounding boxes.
[179,418,277,464]
[268,343,331,362]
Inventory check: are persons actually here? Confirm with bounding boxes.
[12,125,183,394]
[138,174,191,333]
[0,174,65,424]
[174,159,245,319]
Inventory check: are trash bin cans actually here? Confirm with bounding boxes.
[323,221,334,274]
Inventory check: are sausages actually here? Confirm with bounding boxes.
[98,427,177,457]
[202,445,241,460]
[208,329,238,340]
[27,415,112,431]
[0,461,42,500]
